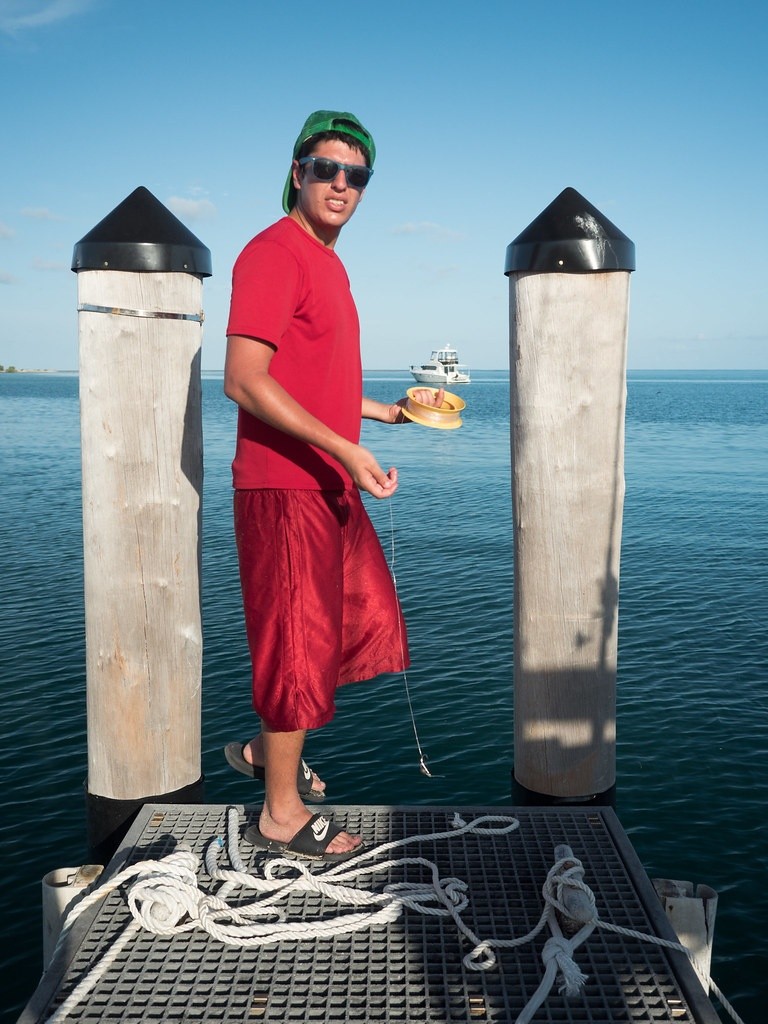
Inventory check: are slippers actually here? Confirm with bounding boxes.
[242,813,366,862]
[224,741,328,803]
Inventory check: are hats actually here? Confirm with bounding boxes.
[281,110,376,215]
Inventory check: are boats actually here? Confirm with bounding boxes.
[410,343,471,383]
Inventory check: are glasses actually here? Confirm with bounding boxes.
[299,156,374,188]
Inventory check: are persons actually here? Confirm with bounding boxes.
[224,109,445,862]
